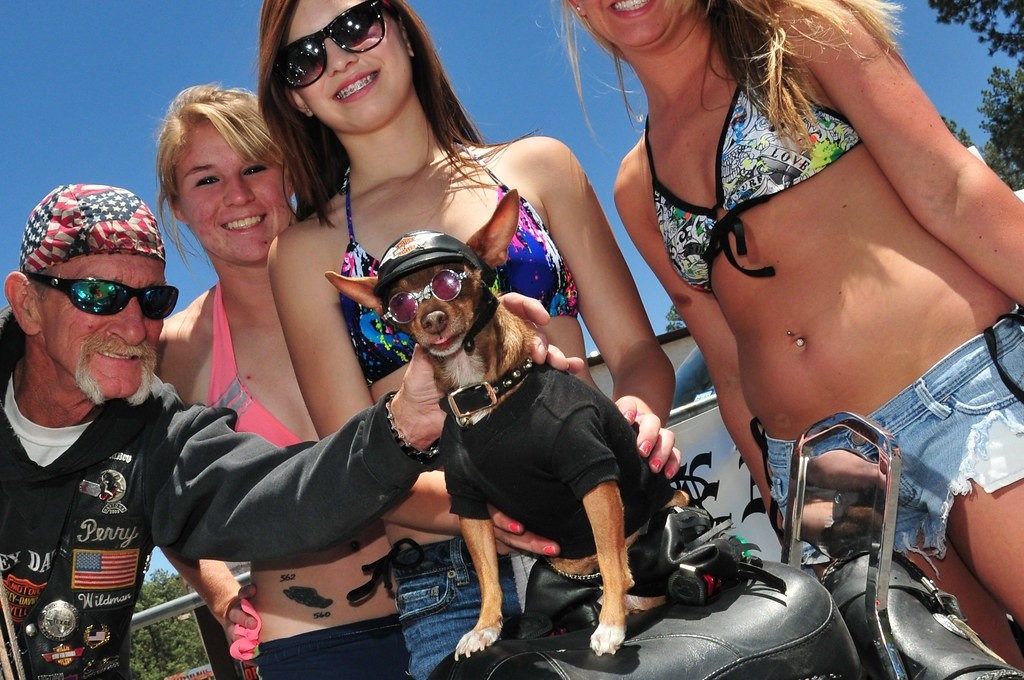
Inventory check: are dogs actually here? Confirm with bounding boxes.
[324,188,691,661]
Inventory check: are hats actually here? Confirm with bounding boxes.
[20,184,167,272]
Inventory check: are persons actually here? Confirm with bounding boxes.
[153,81,411,680]
[555,0,1024,672]
[258,0,681,680]
[0,185,584,680]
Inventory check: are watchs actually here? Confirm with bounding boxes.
[386,393,440,464]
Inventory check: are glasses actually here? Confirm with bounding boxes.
[273,0,385,88]
[383,269,470,323]
[22,273,178,320]
